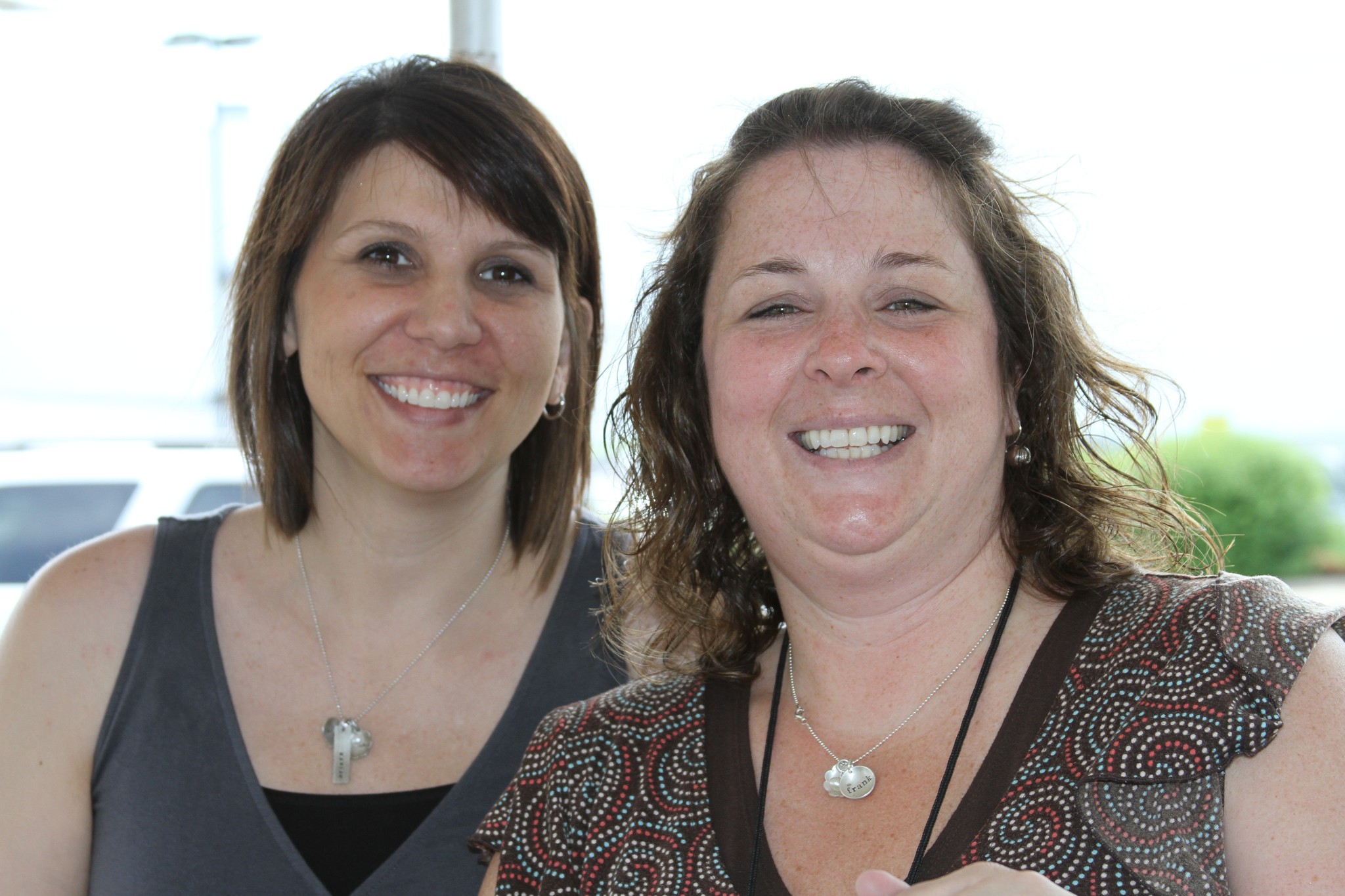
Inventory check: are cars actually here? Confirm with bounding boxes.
[0,435,258,639]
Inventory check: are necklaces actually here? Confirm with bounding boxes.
[295,519,511,786]
[789,589,1013,800]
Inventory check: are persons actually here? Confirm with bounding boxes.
[0,57,726,896]
[470,78,1345,896]
